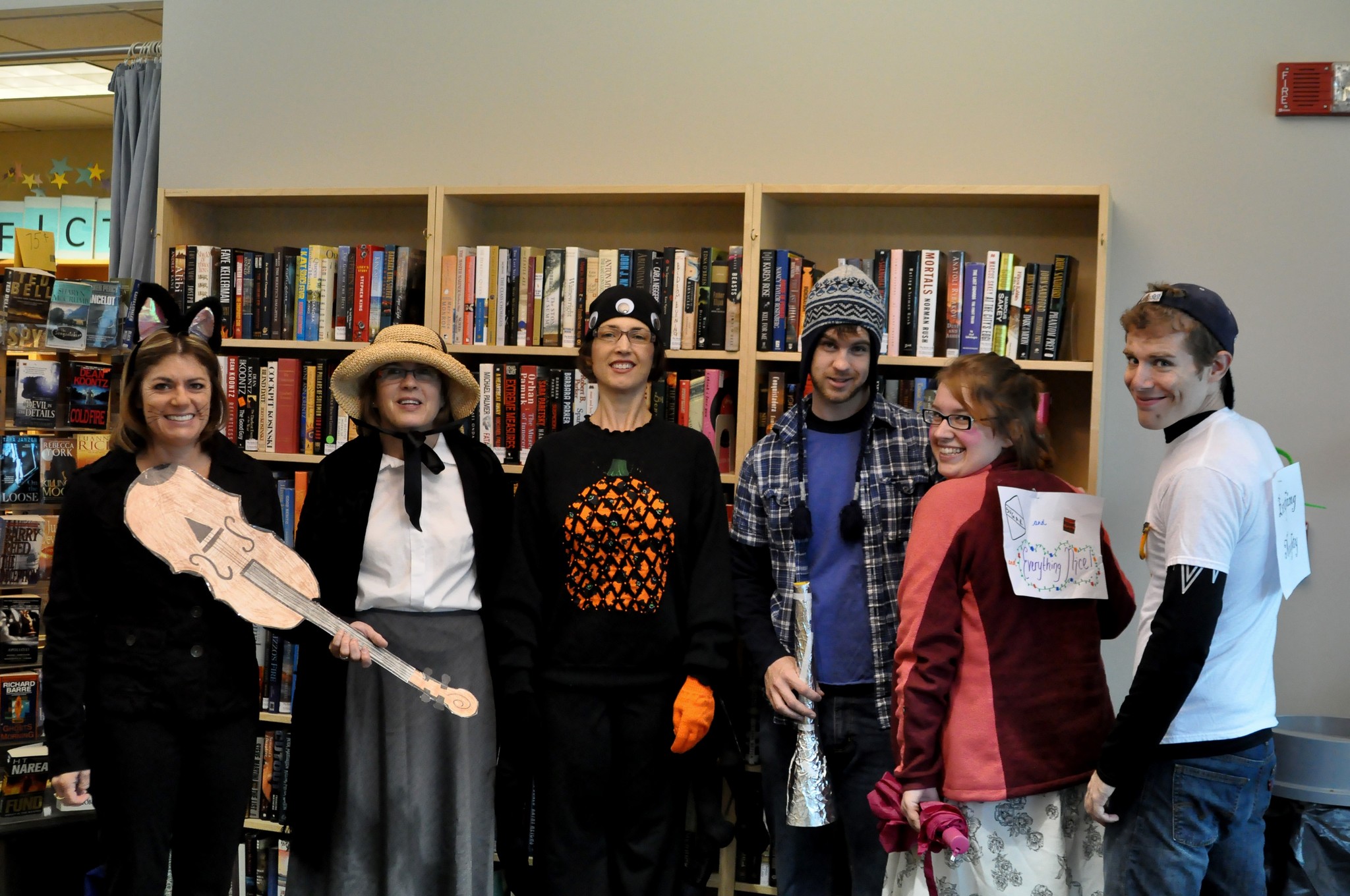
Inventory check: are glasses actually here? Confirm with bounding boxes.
[921,409,1001,430]
[372,367,445,383]
[592,326,657,344]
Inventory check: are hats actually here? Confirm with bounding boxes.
[800,264,884,372]
[1137,283,1239,410]
[588,284,665,358]
[330,324,480,424]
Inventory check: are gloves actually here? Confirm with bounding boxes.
[671,675,715,754]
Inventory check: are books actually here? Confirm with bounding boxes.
[439,245,745,472]
[0,266,131,820]
[756,248,1081,443]
[169,244,425,896]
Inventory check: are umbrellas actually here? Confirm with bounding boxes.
[867,772,969,864]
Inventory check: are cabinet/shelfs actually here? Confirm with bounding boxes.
[1,188,1106,896]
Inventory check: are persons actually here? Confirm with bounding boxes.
[882,348,1136,896]
[729,265,946,896]
[497,287,733,896]
[41,283,283,896]
[1081,280,1286,896]
[285,322,517,896]
